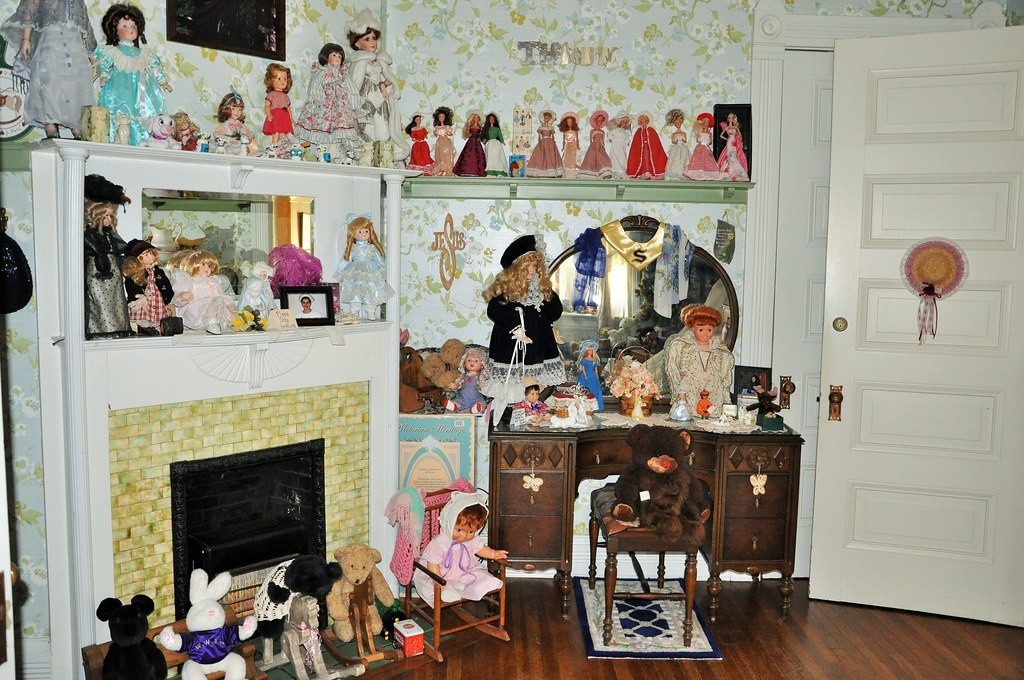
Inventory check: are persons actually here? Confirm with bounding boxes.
[211,8,411,168]
[0,1,173,149]
[669,389,693,422]
[412,491,508,608]
[695,388,713,420]
[406,107,749,181]
[512,380,548,430]
[576,339,606,412]
[442,343,490,415]
[294,293,322,318]
[481,235,567,405]
[668,307,730,419]
[332,215,394,321]
[664,302,723,386]
[83,172,277,333]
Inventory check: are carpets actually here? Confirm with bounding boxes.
[571,576,723,660]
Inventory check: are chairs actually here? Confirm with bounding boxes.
[402,478,513,663]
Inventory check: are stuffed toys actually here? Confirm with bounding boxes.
[399,339,466,414]
[612,425,710,546]
[599,298,670,349]
[745,388,781,416]
[138,111,227,153]
[96,544,395,680]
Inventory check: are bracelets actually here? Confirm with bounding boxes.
[21,37,32,41]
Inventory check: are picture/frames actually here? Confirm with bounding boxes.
[279,286,335,326]
[734,365,772,404]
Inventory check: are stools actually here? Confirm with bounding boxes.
[588,482,705,646]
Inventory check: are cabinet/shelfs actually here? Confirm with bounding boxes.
[487,409,803,624]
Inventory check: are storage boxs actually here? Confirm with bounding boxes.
[758,414,783,430]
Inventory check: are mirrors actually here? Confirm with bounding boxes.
[525,215,740,404]
